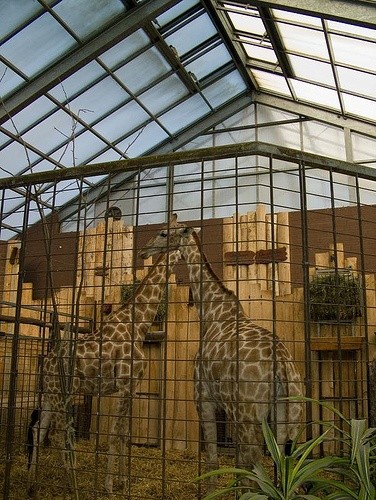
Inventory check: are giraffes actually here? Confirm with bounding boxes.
[136,212,308,500]
[24,227,204,500]
